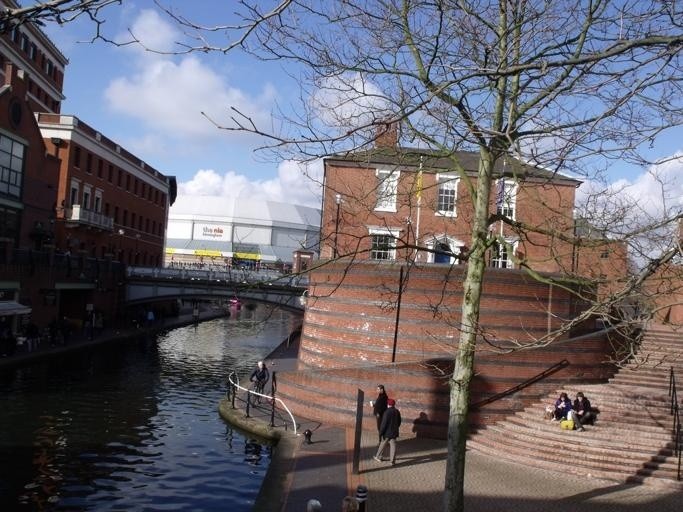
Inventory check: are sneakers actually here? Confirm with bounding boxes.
[390,461,395,466]
[372,455,382,463]
[577,427,585,432]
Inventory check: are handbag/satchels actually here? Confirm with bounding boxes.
[544,407,553,420]
[560,419,575,430]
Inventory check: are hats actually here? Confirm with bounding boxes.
[386,399,395,406]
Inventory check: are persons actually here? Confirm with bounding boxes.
[373,385,388,447]
[306,499,322,511]
[341,495,359,512]
[373,399,401,465]
[551,392,591,432]
[0,320,39,357]
[250,361,269,393]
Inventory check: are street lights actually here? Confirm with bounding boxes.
[334,195,342,259]
[405,218,412,264]
[489,224,493,266]
[572,210,578,273]
